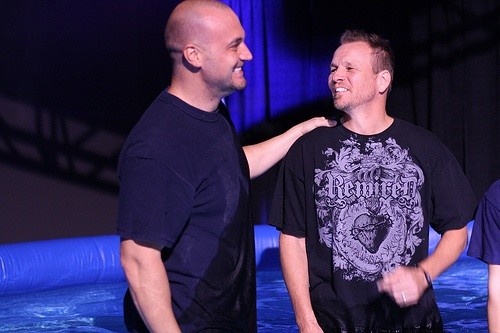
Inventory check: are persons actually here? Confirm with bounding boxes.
[466,177,500,333]
[265,29,479,333]
[114,0,337,333]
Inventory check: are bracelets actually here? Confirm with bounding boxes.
[417,263,434,289]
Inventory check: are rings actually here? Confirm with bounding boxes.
[401,292,407,303]
